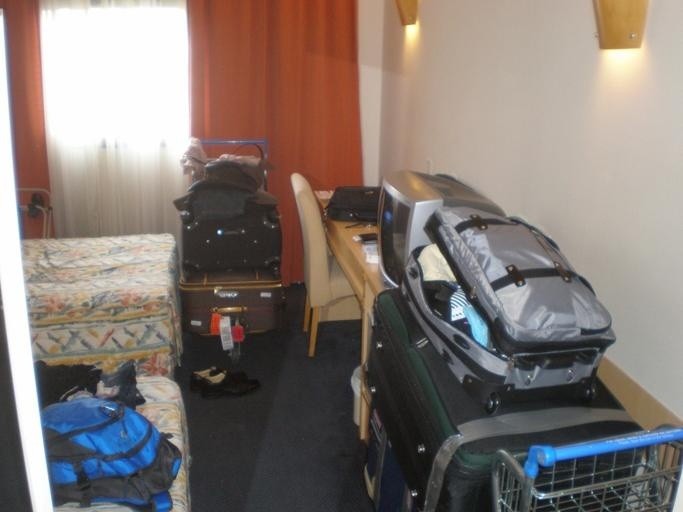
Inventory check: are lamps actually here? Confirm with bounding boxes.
[592,0,648,51]
[397,0,418,26]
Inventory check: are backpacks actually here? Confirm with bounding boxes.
[174,179,279,223]
[398,205,619,420]
[41,397,182,512]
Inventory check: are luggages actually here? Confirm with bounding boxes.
[179,268,283,339]
[180,206,284,282]
[363,290,649,510]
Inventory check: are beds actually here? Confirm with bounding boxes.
[20,230,185,372]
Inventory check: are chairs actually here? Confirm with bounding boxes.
[289,171,362,358]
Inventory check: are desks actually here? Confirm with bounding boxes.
[315,196,399,442]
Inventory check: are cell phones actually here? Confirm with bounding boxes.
[353,233,377,242]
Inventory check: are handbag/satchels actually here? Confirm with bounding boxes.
[276,281,308,337]
[324,187,383,223]
[93,360,146,410]
[203,144,273,194]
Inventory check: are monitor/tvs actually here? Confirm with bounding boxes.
[377,171,506,290]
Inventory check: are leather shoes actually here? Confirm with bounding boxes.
[201,368,262,399]
[191,366,249,394]
